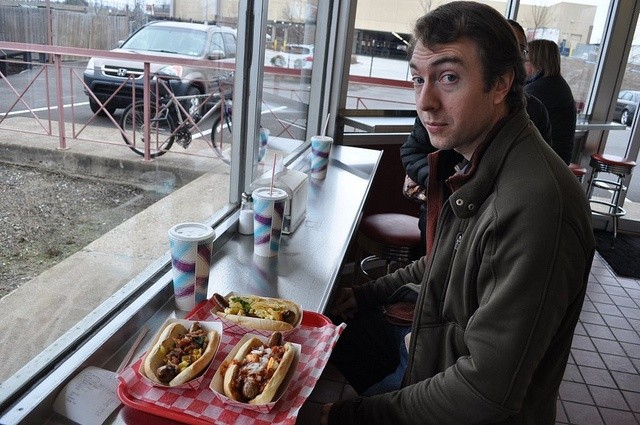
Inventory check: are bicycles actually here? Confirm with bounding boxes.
[120,71,266,168]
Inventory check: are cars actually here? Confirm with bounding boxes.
[281,43,314,69]
[264,34,287,68]
[612,90,640,126]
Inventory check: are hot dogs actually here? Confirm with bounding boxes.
[144,321,220,387]
[213,292,301,331]
[222,330,297,404]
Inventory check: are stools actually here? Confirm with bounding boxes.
[584,154,637,239]
[359,214,427,281]
[568,162,587,185]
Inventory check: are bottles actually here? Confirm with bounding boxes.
[240,193,256,240]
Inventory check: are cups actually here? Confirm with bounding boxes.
[166,221,217,314]
[251,186,288,260]
[310,133,335,182]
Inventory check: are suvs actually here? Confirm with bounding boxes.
[84,19,238,127]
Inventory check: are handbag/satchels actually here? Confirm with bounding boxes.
[403,174,427,203]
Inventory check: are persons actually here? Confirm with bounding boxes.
[321,1,596,425]
[524,39,576,166]
[400,20,554,255]
